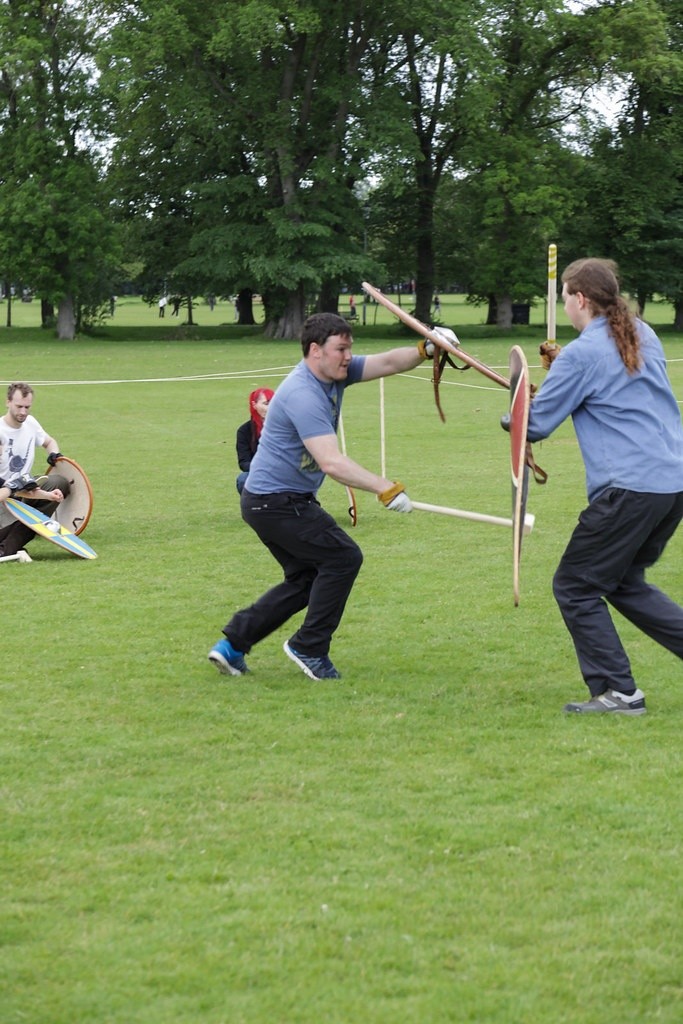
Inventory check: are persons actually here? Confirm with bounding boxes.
[233,294,240,321]
[171,298,181,318]
[206,312,445,680]
[207,295,216,311]
[109,295,116,315]
[526,256,683,718]
[158,297,166,318]
[0,382,70,560]
[349,293,357,316]
[433,296,443,315]
[236,388,276,495]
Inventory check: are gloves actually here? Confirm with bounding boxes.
[3,472,37,497]
[418,326,460,359]
[378,482,413,513]
[47,452,63,467]
[539,341,561,370]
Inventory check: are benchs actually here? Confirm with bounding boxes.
[338,312,360,322]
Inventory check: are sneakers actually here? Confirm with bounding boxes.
[283,639,340,681]
[564,681,647,715]
[208,640,249,676]
